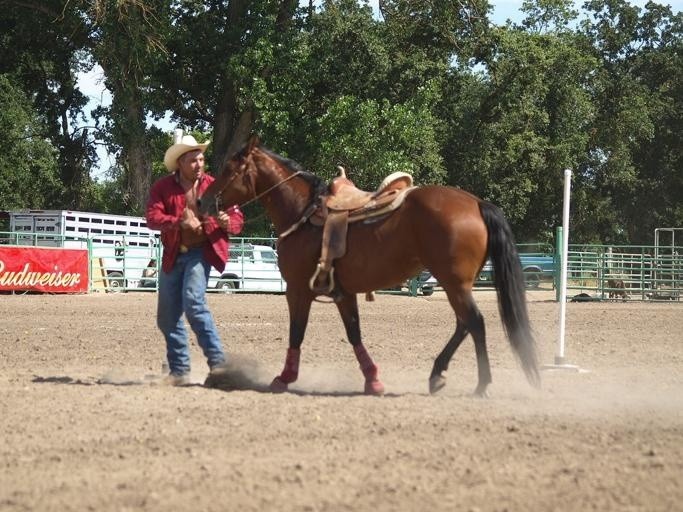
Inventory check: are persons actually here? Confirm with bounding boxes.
[145,135,243,386]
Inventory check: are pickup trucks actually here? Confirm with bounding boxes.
[205,243,288,293]
[473,251,555,291]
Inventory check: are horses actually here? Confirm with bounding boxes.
[608,280,630,302]
[196,132,544,397]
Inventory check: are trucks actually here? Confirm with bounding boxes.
[6,210,167,291]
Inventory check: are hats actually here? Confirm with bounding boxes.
[163,135,210,173]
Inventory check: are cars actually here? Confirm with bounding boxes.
[389,270,438,296]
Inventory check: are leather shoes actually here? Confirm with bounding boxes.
[149,374,190,387]
